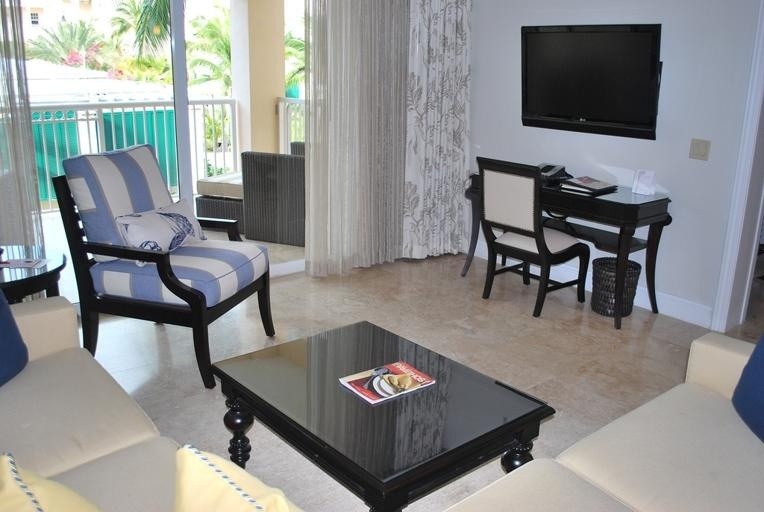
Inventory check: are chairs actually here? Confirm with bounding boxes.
[51,143,276,389]
[195,142,305,247]
[476,156,590,318]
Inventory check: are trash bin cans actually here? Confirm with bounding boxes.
[591,257,641,317]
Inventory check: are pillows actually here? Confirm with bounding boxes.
[62,144,173,264]
[0,453,102,511]
[115,197,207,268]
[0,289,28,386]
[731,327,764,446]
[175,443,303,512]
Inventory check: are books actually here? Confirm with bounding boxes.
[559,176,617,197]
[339,360,436,405]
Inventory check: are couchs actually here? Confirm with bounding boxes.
[441,329,764,512]
[0,289,303,512]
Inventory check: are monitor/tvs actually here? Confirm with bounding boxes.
[521,24,661,140]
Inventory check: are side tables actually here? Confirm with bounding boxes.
[0,244,66,305]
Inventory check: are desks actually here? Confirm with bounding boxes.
[459,173,672,328]
